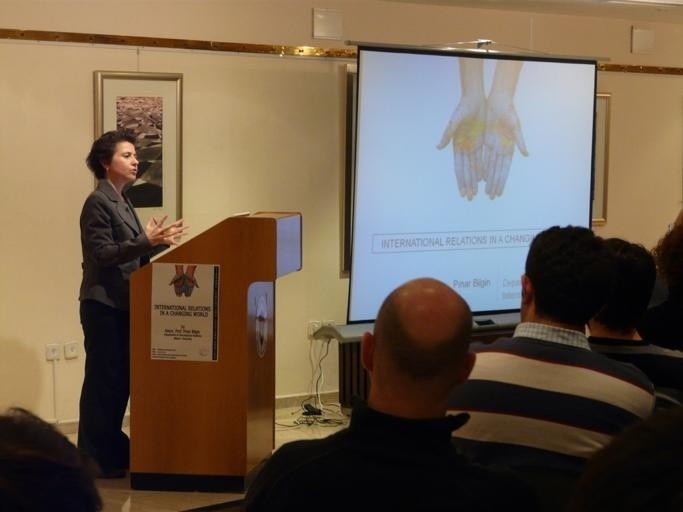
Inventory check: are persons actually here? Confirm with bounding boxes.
[446,224,656,484]
[639,208,683,353]
[436,57,530,202]
[241,276,512,511]
[585,237,676,399]
[0,406,104,512]
[78,130,190,479]
[568,409,683,512]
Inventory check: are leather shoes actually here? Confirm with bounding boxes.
[93,467,127,480]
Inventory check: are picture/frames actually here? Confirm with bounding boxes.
[93,69,184,245]
